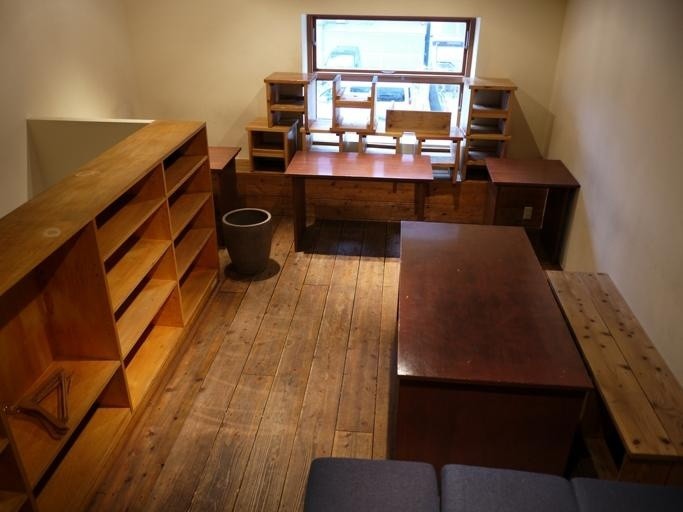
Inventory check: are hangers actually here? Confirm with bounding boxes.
[4,367,74,441]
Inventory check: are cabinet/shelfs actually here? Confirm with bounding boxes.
[0,119,222,512]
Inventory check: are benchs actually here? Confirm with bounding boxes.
[547,269,682,482]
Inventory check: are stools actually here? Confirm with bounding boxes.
[245,72,517,182]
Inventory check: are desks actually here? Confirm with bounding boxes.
[209,147,243,248]
[480,157,580,267]
[284,151,434,252]
[395,219,594,481]
